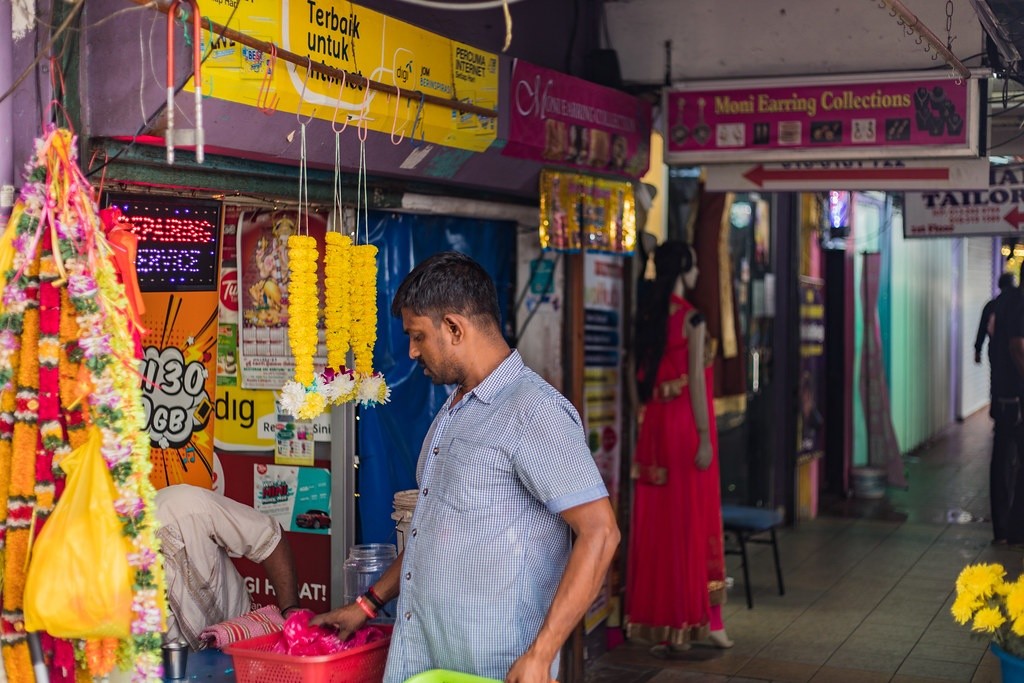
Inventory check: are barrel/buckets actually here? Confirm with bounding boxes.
[387,490,420,556]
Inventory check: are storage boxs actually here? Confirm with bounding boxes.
[220,622,393,683]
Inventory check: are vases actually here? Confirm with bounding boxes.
[991,642,1024,683]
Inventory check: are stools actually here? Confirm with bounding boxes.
[720,504,785,608]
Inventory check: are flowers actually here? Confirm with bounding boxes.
[951,564,1024,657]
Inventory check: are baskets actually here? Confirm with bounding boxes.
[222,624,393,683]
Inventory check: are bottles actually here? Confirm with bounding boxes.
[343,543,398,625]
[258,475,289,504]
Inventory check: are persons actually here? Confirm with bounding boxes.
[149,484,303,652]
[975,261,1024,551]
[624,238,735,649]
[309,254,621,683]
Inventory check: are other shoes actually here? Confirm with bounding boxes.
[1008,543,1024,552]
[991,538,1007,546]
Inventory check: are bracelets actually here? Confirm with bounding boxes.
[281,605,300,616]
[356,588,385,619]
